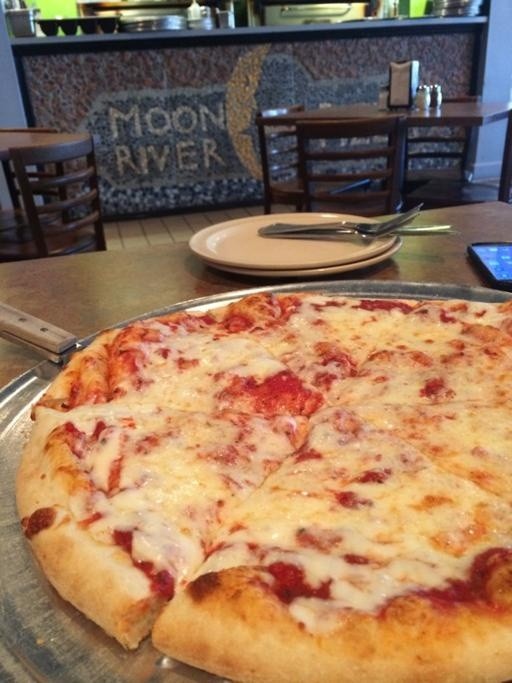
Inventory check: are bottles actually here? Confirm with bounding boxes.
[418,84,444,111]
[378,85,390,110]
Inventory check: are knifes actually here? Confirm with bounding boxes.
[388,223,459,235]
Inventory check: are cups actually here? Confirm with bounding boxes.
[34,17,120,35]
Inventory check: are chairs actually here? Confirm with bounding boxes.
[0,136,107,262]
[402,96,481,184]
[255,104,372,213]
[0,128,66,230]
[296,113,404,214]
[406,108,512,207]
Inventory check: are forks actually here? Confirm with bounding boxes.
[259,198,425,240]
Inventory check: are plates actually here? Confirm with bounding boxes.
[123,14,191,32]
[436,0,485,18]
[189,212,403,278]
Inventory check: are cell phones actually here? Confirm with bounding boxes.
[468,242,512,293]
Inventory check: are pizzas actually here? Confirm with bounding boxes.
[15,291,512,683]
